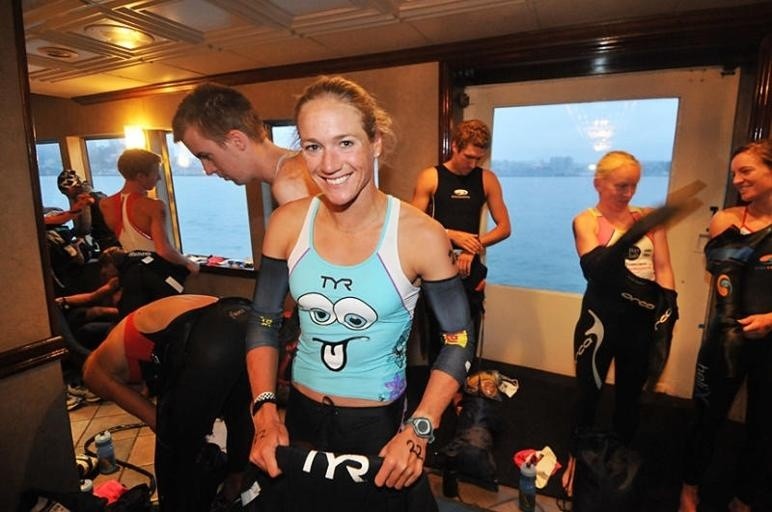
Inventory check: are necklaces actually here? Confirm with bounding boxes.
[275,148,290,178]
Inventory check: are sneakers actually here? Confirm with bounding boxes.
[62,380,106,412]
[75,453,99,479]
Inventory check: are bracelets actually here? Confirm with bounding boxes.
[252,391,280,417]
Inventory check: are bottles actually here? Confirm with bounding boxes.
[95,431,117,474]
[519,462,537,512]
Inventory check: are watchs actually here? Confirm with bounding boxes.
[401,416,436,446]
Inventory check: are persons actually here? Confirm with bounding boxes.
[53,244,126,411]
[409,116,511,420]
[45,167,121,249]
[562,149,678,497]
[171,79,325,352]
[81,293,250,507]
[676,133,772,512]
[245,72,477,491]
[99,149,201,280]
